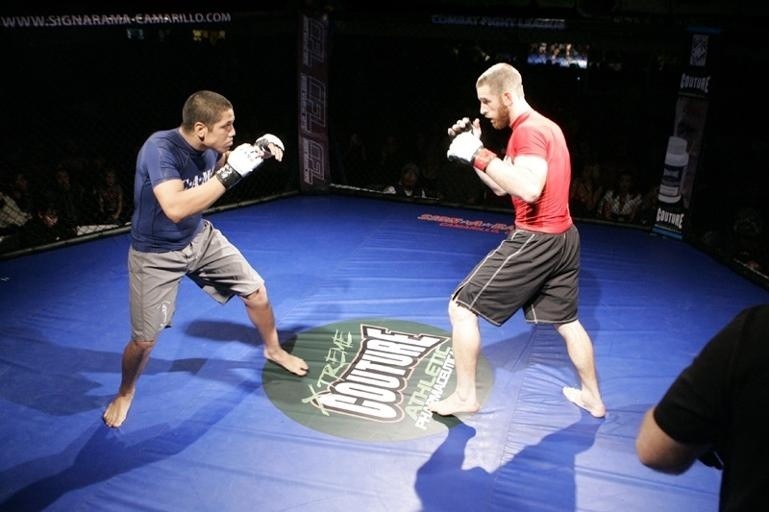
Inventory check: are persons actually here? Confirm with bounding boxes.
[570,163,767,275]
[102,89,310,429]
[527,40,591,67]
[384,163,424,198]
[634,301,767,511]
[3,134,132,255]
[426,62,607,419]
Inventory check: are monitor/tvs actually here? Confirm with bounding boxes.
[524,42,591,70]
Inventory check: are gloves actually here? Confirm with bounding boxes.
[255,133,285,159]
[444,131,497,174]
[450,116,483,140]
[215,142,266,190]
